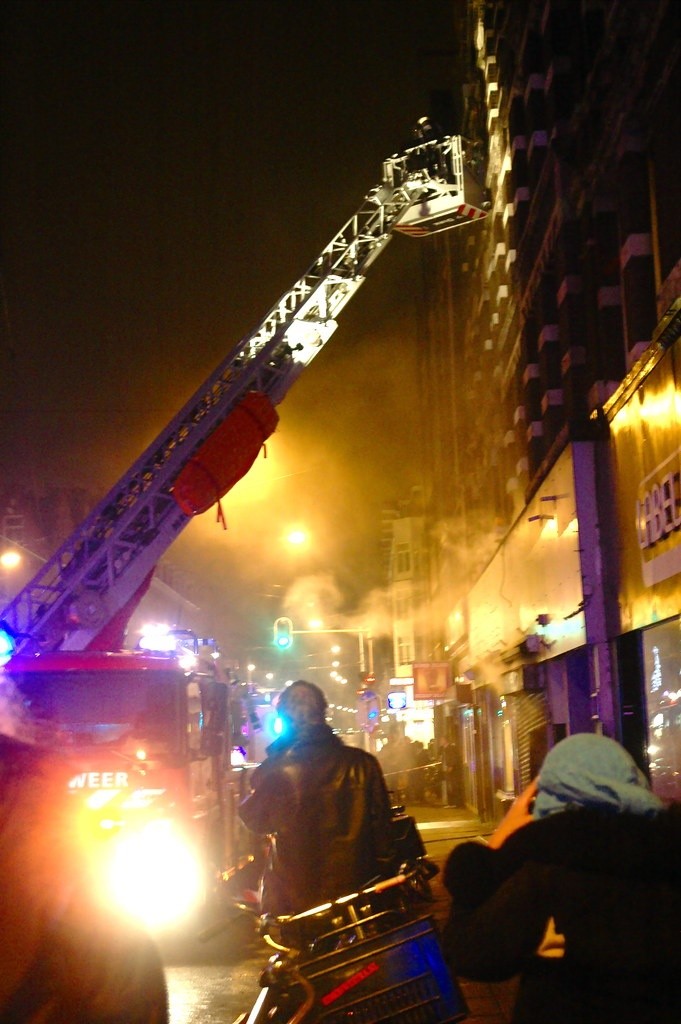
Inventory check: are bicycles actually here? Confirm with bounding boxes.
[241,789,473,1024]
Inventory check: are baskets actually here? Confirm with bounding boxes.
[271,910,470,1024]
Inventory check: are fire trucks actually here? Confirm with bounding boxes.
[0,104,493,953]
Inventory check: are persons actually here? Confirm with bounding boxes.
[441,732,681,1024]
[411,117,447,170]
[375,733,460,810]
[238,680,401,942]
[0,731,171,1024]
